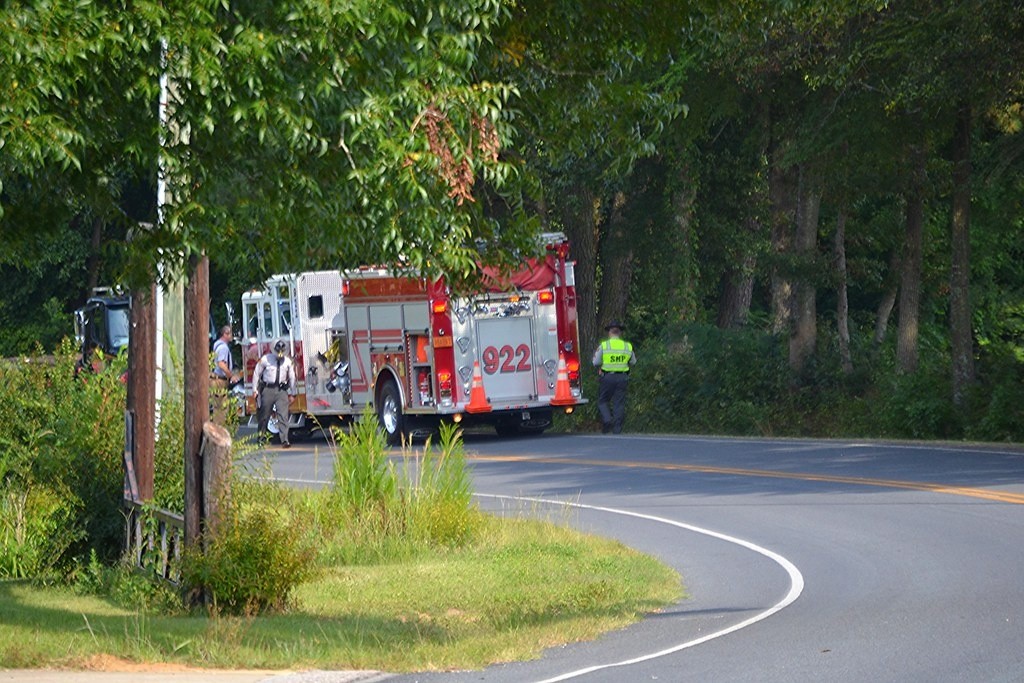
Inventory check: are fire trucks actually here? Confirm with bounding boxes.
[226,231,590,447]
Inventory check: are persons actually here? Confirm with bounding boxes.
[210,326,239,427]
[251,340,297,447]
[592,320,637,434]
[86,341,117,375]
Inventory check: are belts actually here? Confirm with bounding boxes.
[601,369,629,375]
[210,376,227,380]
[262,382,282,388]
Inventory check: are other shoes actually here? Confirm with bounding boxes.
[257,441,266,447]
[602,422,611,434]
[283,442,291,449]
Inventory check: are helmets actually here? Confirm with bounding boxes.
[604,321,626,331]
[269,338,289,357]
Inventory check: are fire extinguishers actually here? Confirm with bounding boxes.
[418,368,431,406]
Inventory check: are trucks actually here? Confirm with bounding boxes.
[73,284,217,381]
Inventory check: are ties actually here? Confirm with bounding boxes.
[275,359,280,386]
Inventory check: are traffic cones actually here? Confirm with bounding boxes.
[463,360,493,414]
[548,350,578,405]
